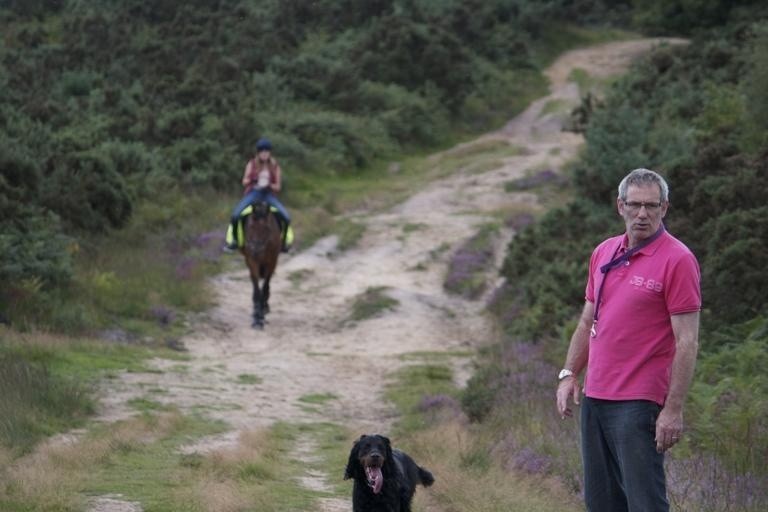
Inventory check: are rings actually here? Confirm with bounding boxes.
[672,436,678,439]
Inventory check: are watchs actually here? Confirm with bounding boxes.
[558,369,577,380]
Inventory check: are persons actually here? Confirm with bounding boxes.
[555,168,702,512]
[222,140,294,255]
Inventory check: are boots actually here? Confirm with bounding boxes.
[282,223,290,252]
[228,218,238,250]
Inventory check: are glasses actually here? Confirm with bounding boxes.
[622,198,663,209]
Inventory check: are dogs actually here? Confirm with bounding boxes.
[343,434,436,511]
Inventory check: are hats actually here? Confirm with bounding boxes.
[256,139,272,149]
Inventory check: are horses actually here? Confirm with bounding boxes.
[236,201,287,330]
